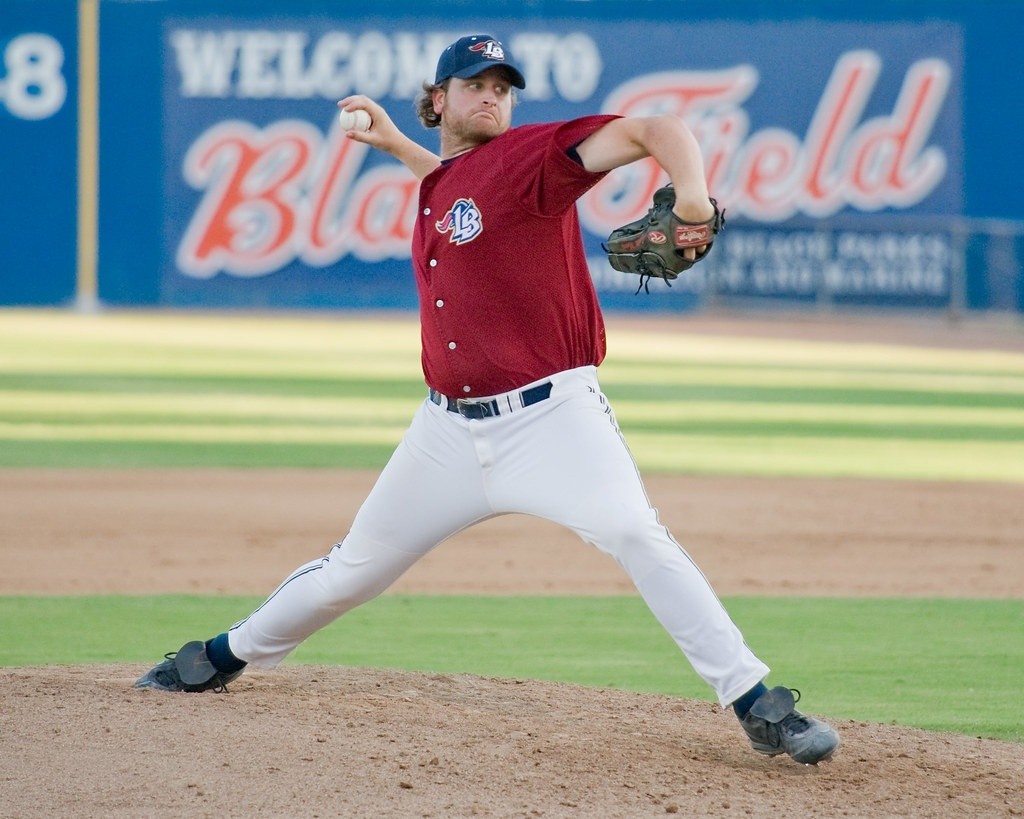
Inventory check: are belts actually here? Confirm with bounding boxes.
[427,382,551,420]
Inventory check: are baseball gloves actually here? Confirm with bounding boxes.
[603,182,728,280]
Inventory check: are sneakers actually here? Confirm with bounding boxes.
[735,684,838,763]
[129,627,243,690]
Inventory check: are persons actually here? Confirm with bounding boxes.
[132,33,843,768]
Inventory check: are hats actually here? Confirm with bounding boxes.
[434,35,526,92]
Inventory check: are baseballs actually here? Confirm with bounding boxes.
[339,105,372,138]
